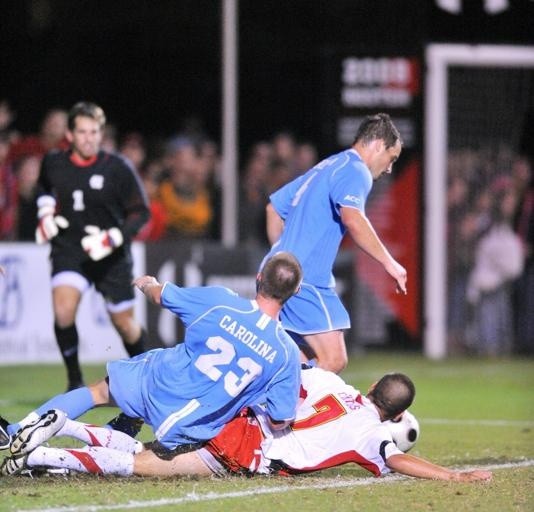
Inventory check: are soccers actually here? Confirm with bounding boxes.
[383,409,419,453]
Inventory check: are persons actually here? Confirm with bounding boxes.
[0,366,493,483]
[253,113,409,375]
[30,100,152,395]
[0,252,303,451]
[0,98,533,360]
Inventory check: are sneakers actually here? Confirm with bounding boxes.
[0,416,12,451]
[9,409,66,455]
[0,454,36,478]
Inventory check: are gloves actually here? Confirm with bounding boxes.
[33,194,69,246]
[81,224,126,261]
